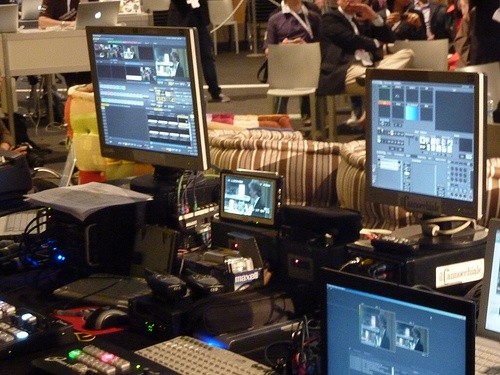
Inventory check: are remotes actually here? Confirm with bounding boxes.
[369,234,418,250]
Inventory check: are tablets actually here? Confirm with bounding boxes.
[218,169,278,224]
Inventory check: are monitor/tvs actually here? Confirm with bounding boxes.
[313,267,476,375]
[365,67,491,250]
[477,216,500,340]
[83,26,208,187]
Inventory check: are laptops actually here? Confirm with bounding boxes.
[66,0,121,30]
[0,3,19,34]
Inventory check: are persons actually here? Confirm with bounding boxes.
[265,0,500,132]
[244,180,265,217]
[167,0,232,103]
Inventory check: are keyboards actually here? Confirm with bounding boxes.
[135,334,279,375]
[0,293,74,358]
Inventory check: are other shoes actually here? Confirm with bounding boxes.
[356,73,365,87]
[302,115,313,126]
[212,93,231,102]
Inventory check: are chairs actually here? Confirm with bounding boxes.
[393,39,452,71]
[206,114,294,133]
[335,138,500,230]
[453,61,500,113]
[207,130,345,207]
[264,41,328,141]
[208,0,239,55]
[65,85,154,180]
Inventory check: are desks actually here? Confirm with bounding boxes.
[0,22,127,146]
